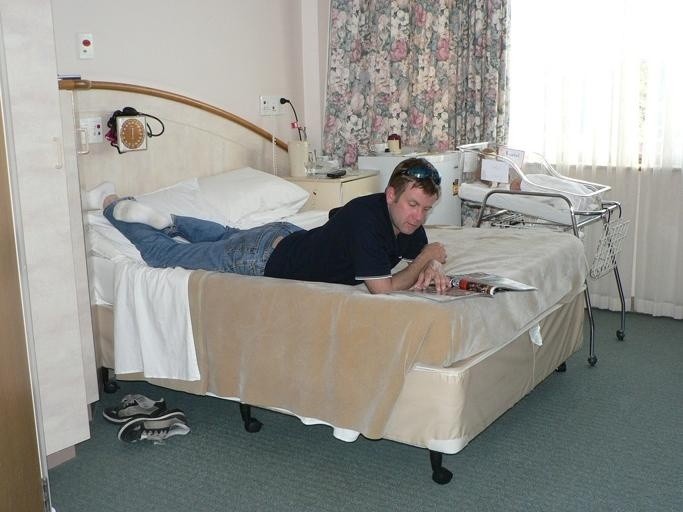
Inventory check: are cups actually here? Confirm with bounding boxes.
[370,144,386,152]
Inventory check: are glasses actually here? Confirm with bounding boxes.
[398,165,443,188]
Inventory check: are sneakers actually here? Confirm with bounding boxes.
[103,393,169,424]
[117,408,190,446]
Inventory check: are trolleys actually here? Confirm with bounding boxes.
[454,141,634,369]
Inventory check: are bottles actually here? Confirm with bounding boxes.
[387,134,402,155]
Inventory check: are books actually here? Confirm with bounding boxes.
[391,269,540,304]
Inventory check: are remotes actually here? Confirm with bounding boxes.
[326,169,347,177]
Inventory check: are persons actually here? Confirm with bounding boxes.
[79,156,454,295]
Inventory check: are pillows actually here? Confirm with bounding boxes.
[82,166,309,264]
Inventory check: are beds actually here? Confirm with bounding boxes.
[56,74,588,486]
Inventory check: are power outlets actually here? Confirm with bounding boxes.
[259,95,287,117]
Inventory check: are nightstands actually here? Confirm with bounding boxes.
[281,168,381,212]
